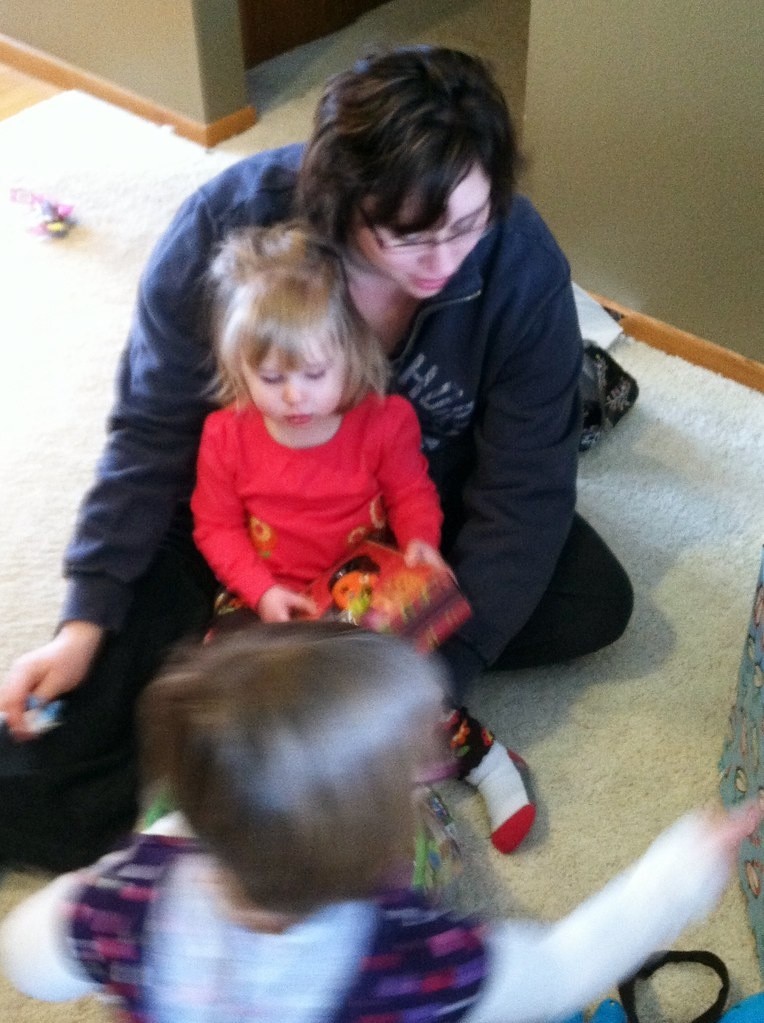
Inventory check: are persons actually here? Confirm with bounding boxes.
[189,217,541,855]
[7,34,638,879]
[12,611,764,1023]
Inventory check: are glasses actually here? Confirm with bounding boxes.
[353,197,502,253]
